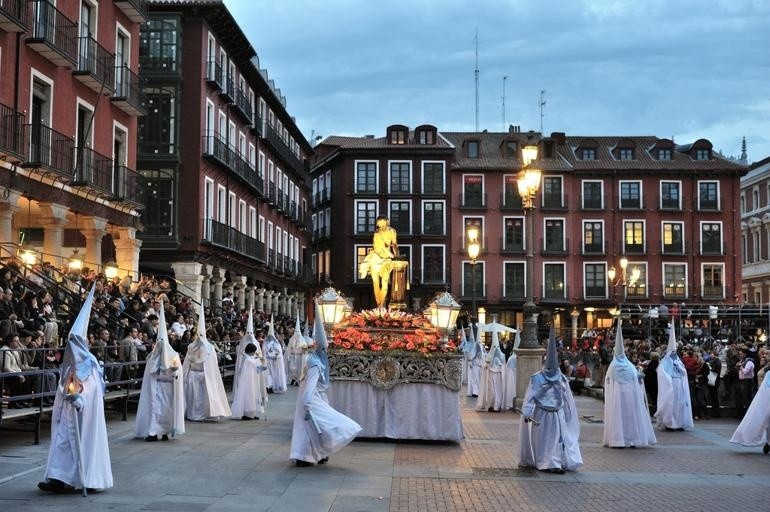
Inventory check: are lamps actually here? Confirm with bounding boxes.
[22,197,36,253]
[68,211,83,262]
[104,220,119,269]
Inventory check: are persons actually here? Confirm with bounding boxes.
[38,280,114,492]
[132,297,186,442]
[283,310,311,386]
[728,371,770,454]
[604,318,658,448]
[262,313,287,393]
[0,261,230,416]
[223,293,313,341]
[356,217,399,306]
[290,302,363,464]
[515,324,583,474]
[458,323,521,412]
[182,299,232,422]
[654,316,693,431]
[232,304,269,421]
[564,302,770,421]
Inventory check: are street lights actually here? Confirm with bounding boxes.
[463,221,480,331]
[606,257,641,303]
[513,141,545,414]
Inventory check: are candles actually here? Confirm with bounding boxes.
[69,383,74,394]
[174,358,176,367]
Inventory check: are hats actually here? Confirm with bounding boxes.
[668,315,677,352]
[461,316,500,351]
[313,298,329,349]
[615,316,625,357]
[69,279,97,339]
[513,322,521,353]
[156,298,309,345]
[546,319,558,368]
